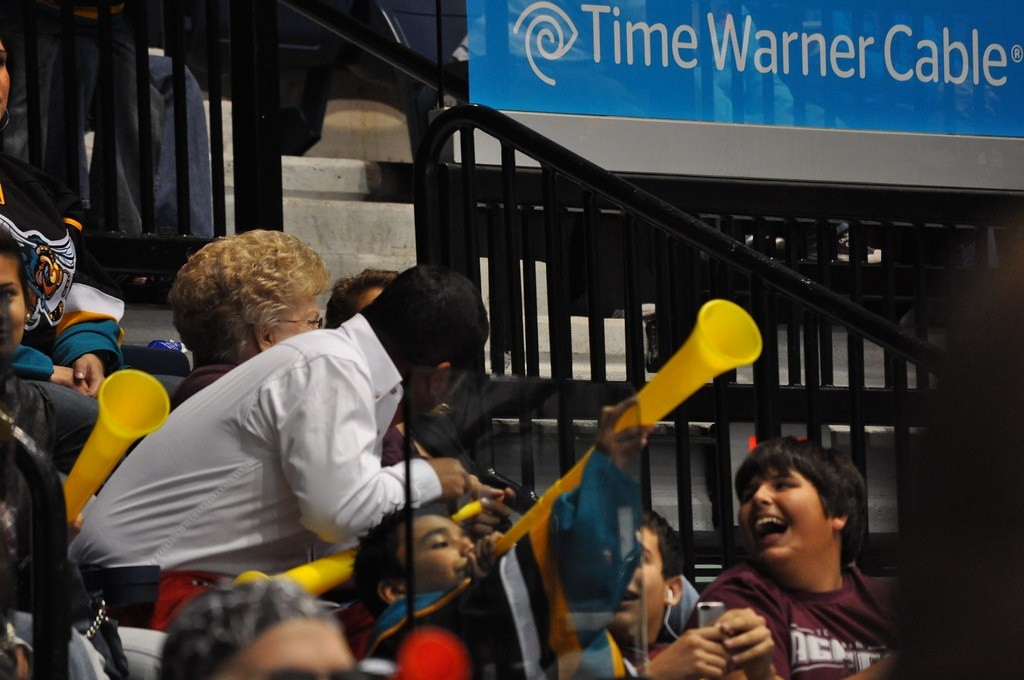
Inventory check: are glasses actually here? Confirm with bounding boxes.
[266,318,323,343]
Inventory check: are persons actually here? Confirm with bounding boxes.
[326,268,514,534]
[352,398,659,680]
[686,434,901,680]
[0,0,357,680]
[608,506,783,680]
[68,263,515,633]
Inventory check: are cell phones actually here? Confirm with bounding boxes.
[697,601,725,629]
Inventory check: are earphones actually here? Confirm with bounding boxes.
[668,589,673,604]
